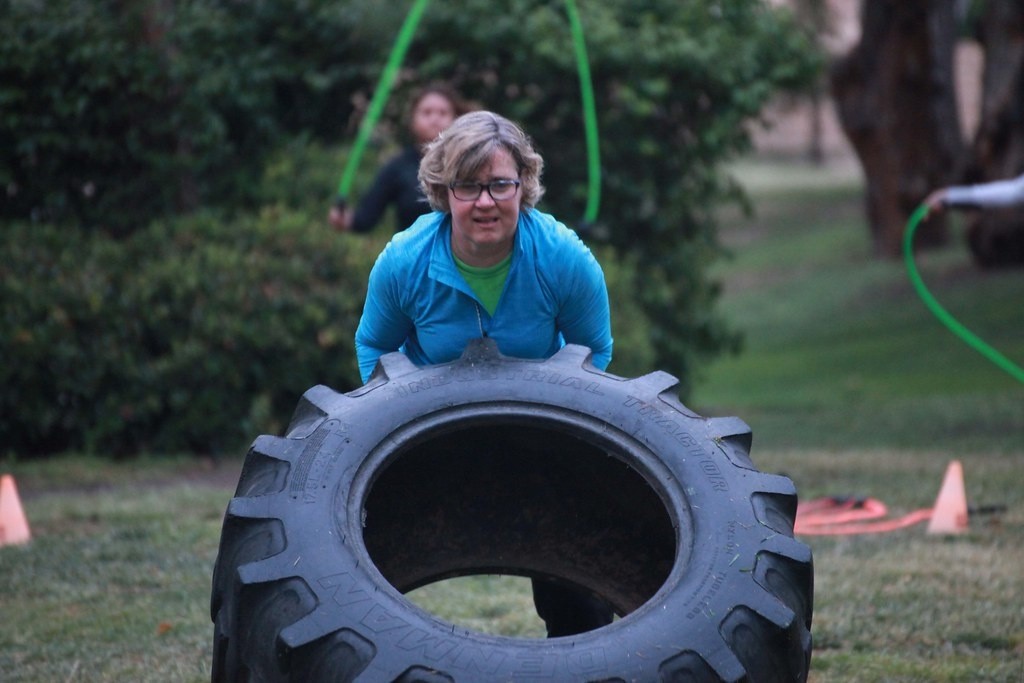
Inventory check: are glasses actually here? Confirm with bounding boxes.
[449,177,520,202]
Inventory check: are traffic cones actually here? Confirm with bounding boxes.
[925,460,969,534]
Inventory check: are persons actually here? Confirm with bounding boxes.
[925,175,1024,221]
[355,110,615,638]
[328,81,465,231]
